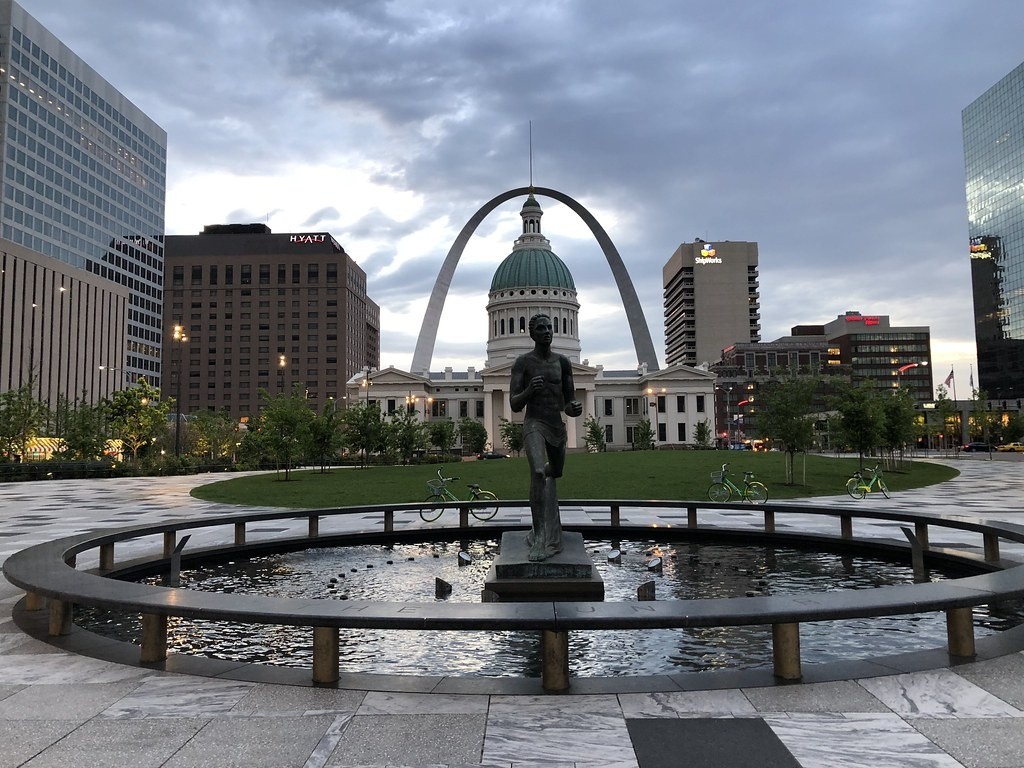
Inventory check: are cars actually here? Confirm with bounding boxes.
[477,451,511,461]
[997,442,1024,452]
[963,442,997,453]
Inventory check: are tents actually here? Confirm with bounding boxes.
[0,438,124,464]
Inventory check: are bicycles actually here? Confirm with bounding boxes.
[846,460,891,499]
[420,466,499,522]
[707,463,769,505]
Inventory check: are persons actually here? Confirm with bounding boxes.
[509,314,582,561]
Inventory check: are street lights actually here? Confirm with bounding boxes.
[330,396,347,411]
[173,325,189,460]
[362,371,373,406]
[280,354,286,393]
[648,388,666,445]
[98,366,132,388]
[715,387,733,450]
[406,390,419,420]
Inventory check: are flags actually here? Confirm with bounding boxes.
[945,370,953,387]
[970,371,973,386]
